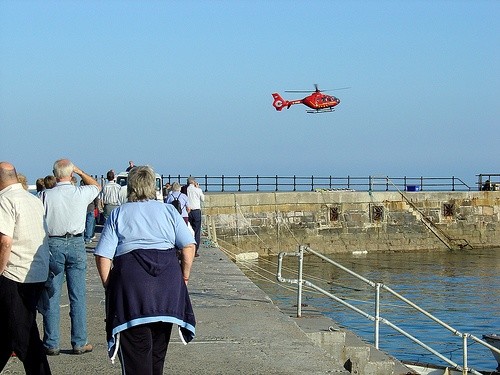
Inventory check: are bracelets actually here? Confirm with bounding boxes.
[182,275,189,281]
[80,171,84,176]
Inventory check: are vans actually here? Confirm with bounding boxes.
[116,172,164,201]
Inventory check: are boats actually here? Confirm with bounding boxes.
[481,333,500,363]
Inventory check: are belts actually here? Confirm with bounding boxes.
[51,233,82,238]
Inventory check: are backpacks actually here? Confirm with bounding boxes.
[171,193,185,214]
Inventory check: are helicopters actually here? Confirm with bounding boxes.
[271,82,351,114]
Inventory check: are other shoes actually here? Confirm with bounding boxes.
[91,236,96,240]
[195,253,199,257]
[73,345,92,354]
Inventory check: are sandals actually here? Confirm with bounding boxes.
[47,348,60,356]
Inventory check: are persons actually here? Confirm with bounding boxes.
[90,194,102,239]
[17,173,28,190]
[36,159,102,356]
[100,171,125,225]
[93,165,197,375]
[126,161,137,174]
[80,180,99,244]
[187,176,204,257]
[36,178,46,192]
[180,179,188,196]
[110,169,115,174]
[36,175,57,197]
[118,185,128,205]
[163,183,171,203]
[165,182,190,257]
[71,177,77,183]
[0,162,52,375]
